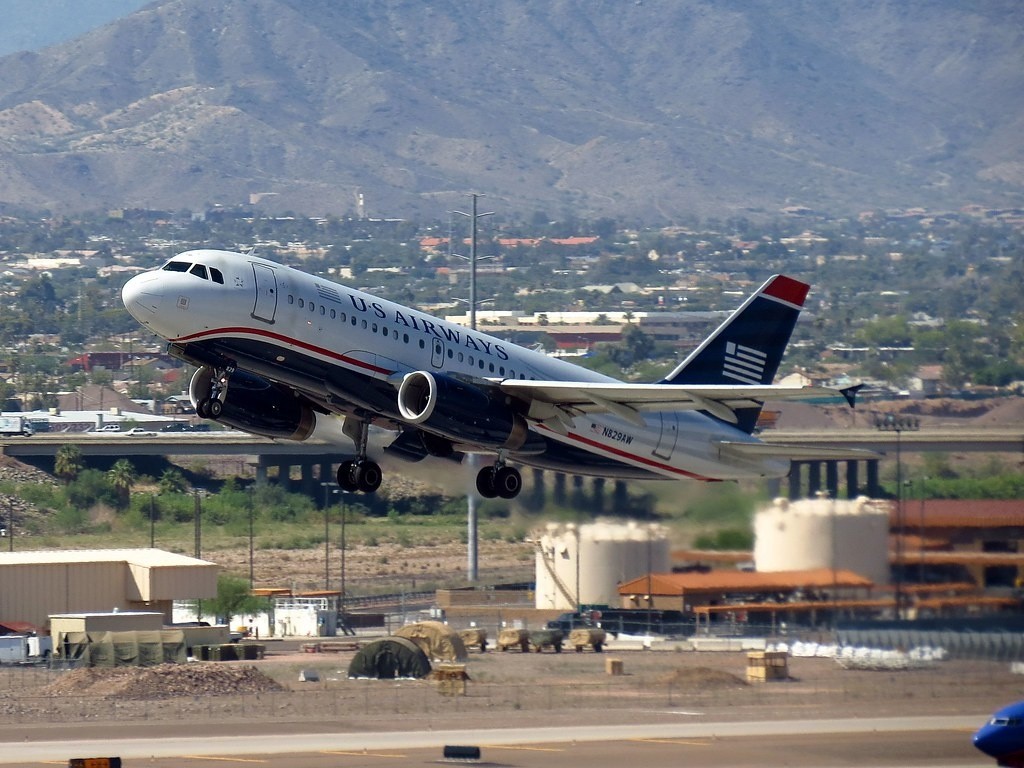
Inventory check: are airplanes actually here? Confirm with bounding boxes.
[120,245,892,500]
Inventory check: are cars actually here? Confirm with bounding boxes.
[159,422,185,432]
[126,427,144,432]
[182,423,211,432]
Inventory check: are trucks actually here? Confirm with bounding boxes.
[0,415,35,437]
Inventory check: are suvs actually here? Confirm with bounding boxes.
[95,424,121,432]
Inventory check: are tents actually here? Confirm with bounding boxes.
[348,634,432,678]
[50,629,188,667]
[391,620,469,664]
